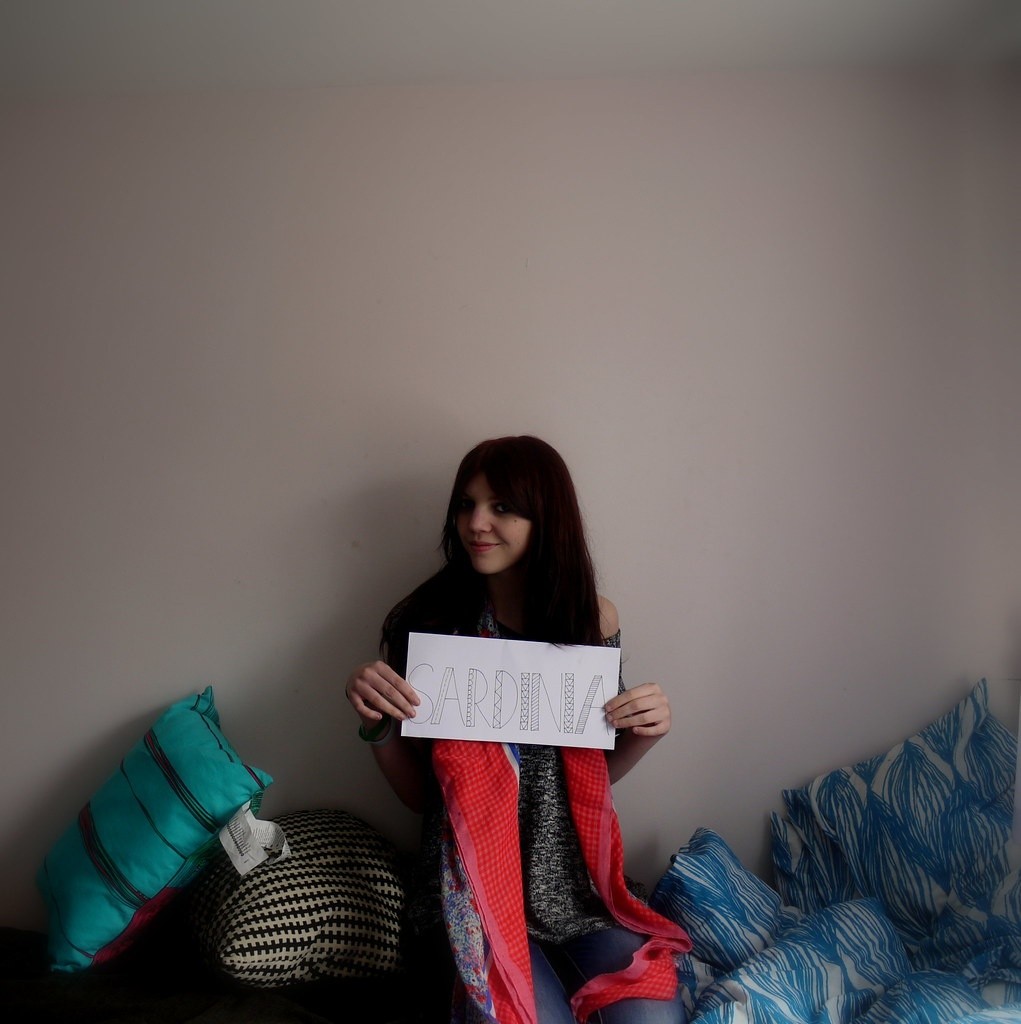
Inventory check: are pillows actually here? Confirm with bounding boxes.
[30,686,273,973]
[184,806,436,988]
[783,677,1021,971]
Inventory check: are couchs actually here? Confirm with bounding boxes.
[0,925,343,1024]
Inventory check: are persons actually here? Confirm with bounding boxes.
[346,436,687,1024]
